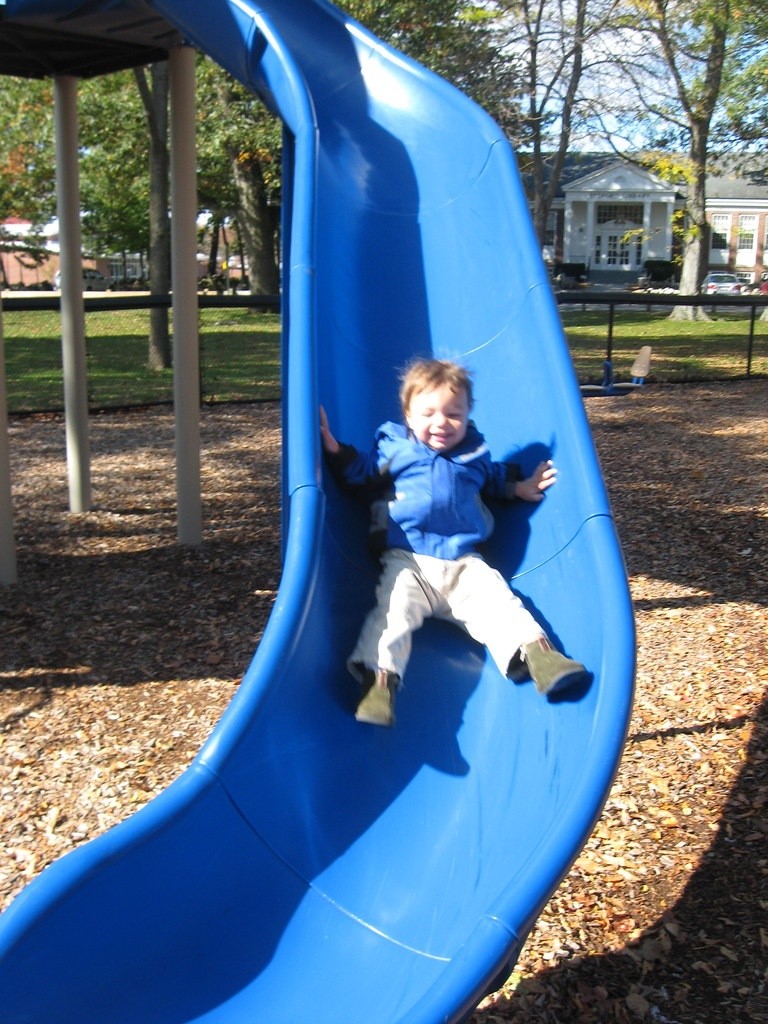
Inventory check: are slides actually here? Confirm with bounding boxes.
[0,0,638,1024]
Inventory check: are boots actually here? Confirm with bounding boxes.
[523,635,591,697]
[352,679,401,729]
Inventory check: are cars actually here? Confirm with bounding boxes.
[701,274,743,296]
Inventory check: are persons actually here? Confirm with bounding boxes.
[319,357,590,726]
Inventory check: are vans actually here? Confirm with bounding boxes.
[53,266,108,291]
